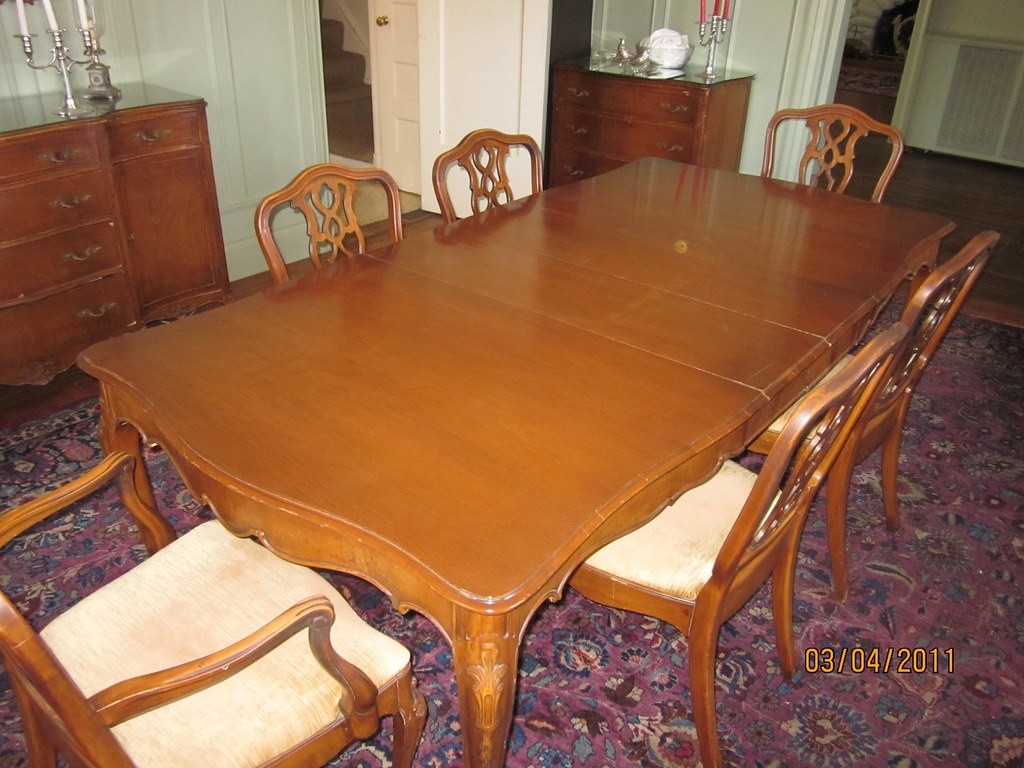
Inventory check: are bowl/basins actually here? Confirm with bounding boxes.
[636,44,695,69]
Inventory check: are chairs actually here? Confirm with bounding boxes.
[255,163,404,279]
[0,454,426,768]
[565,321,907,768]
[759,104,902,202]
[748,230,1003,591]
[430,128,544,227]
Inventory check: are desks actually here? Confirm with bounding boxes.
[79,159,953,768]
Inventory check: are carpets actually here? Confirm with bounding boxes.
[837,65,901,98]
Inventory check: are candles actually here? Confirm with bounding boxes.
[77,0,90,28]
[16,0,28,34]
[713,0,719,16]
[701,0,705,22]
[43,0,59,30]
[723,0,730,19]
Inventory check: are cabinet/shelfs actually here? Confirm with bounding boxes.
[0,82,233,390]
[549,61,754,187]
